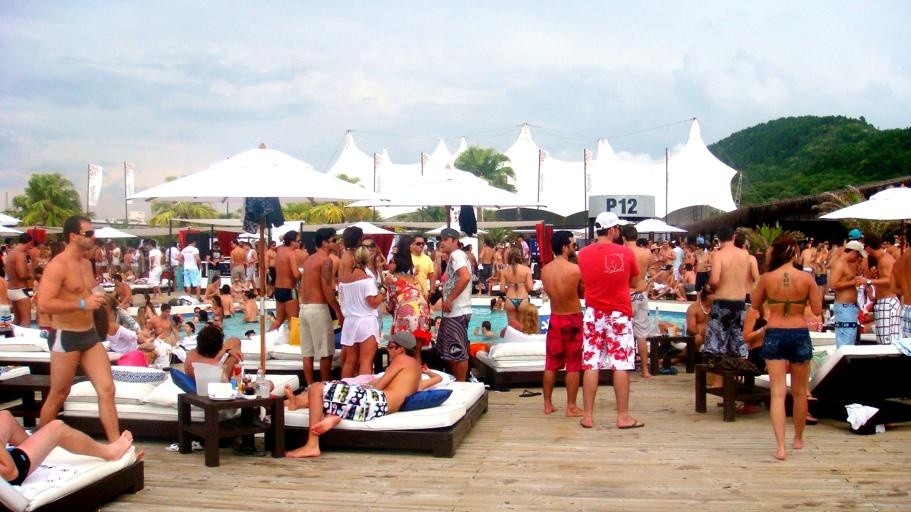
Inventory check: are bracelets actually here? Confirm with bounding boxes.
[80,300,85,311]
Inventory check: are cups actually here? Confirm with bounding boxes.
[259,383,270,399]
[92,286,106,298]
[855,276,861,288]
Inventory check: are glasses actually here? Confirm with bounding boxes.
[415,241,425,246]
[328,238,337,244]
[566,243,577,248]
[77,230,95,238]
[295,239,301,243]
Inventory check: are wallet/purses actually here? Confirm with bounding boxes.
[429,291,442,306]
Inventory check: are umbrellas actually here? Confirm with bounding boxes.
[125,142,390,378]
[0,225,24,240]
[636,220,687,245]
[428,225,486,234]
[819,188,911,245]
[345,164,547,230]
[333,221,396,236]
[0,212,22,225]
[91,226,136,249]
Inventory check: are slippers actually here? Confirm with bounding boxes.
[617,419,647,427]
[580,419,595,428]
[807,414,817,424]
[807,395,817,401]
[165,442,180,453]
[519,388,541,398]
[192,441,204,450]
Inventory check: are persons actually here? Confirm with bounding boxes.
[92,241,163,297]
[477,238,493,295]
[33,215,145,461]
[702,222,751,407]
[686,284,715,364]
[186,286,275,338]
[382,251,431,364]
[502,303,539,336]
[284,358,443,411]
[431,229,473,382]
[362,239,392,338]
[181,324,246,389]
[473,233,542,265]
[285,329,422,458]
[0,229,70,329]
[575,212,647,428]
[750,233,821,459]
[830,240,864,349]
[244,329,255,338]
[539,231,588,418]
[268,231,302,332]
[798,236,870,291]
[500,246,533,334]
[472,263,507,295]
[99,306,187,373]
[1,408,134,486]
[337,244,395,379]
[855,235,901,348]
[855,230,911,257]
[166,241,278,296]
[406,233,436,304]
[890,228,911,347]
[635,230,721,303]
[615,225,654,378]
[298,225,343,383]
[388,241,478,280]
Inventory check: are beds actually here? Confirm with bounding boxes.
[1,367,30,403]
[652,289,697,302]
[63,375,299,446]
[472,340,615,393]
[239,346,342,389]
[263,382,489,458]
[106,278,174,295]
[491,279,542,296]
[184,277,231,294]
[740,346,911,417]
[0,444,144,512]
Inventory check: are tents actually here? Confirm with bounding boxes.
[377,222,543,231]
[167,217,304,230]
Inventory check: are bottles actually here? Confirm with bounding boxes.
[653,307,661,333]
[256,369,265,396]
[681,324,686,334]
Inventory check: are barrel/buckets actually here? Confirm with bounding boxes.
[194,364,223,396]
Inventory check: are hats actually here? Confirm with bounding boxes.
[592,211,628,231]
[383,332,417,351]
[440,228,460,239]
[650,243,661,249]
[848,229,863,240]
[845,240,867,258]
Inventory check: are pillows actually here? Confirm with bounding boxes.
[10,324,49,338]
[399,390,452,413]
[491,343,547,362]
[111,367,167,386]
[64,381,154,406]
[169,368,197,394]
[1,338,50,352]
[810,332,835,345]
[242,340,271,361]
[268,345,340,360]
[145,380,184,408]
